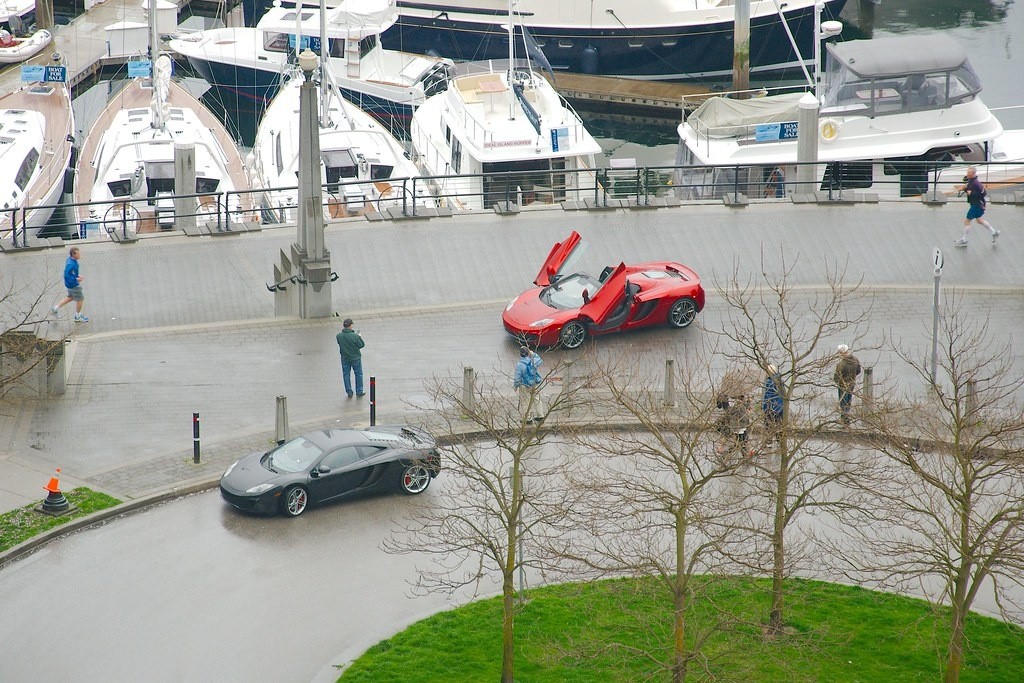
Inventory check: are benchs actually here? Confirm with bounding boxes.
[837,81,903,112]
[456,72,500,103]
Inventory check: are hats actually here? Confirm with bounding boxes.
[837,344,848,352]
[343,318,353,326]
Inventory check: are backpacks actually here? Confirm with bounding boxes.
[520,361,542,385]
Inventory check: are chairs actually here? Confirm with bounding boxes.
[901,74,937,108]
[478,81,506,112]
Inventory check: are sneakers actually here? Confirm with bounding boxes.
[954,238,968,247]
[992,231,1000,244]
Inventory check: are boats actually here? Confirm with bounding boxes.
[410,0,617,212]
[0,35,77,241]
[71,0,261,241]
[168,0,458,136]
[0,0,36,34]
[177,0,848,85]
[246,0,439,223]
[670,0,1024,200]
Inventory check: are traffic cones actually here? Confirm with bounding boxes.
[43,467,62,492]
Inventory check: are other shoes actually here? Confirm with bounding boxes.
[50,309,57,318]
[836,418,849,424]
[357,392,366,397]
[534,417,543,422]
[520,420,533,424]
[75,315,88,322]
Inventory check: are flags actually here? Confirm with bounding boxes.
[522,27,555,81]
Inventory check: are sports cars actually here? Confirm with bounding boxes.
[218,423,441,517]
[502,230,707,351]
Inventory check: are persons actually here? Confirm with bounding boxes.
[51,248,88,322]
[763,365,785,454]
[953,167,1000,246]
[513,346,544,424]
[834,344,861,428]
[336,319,366,397]
[716,389,758,460]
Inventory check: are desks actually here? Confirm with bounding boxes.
[856,88,909,97]
